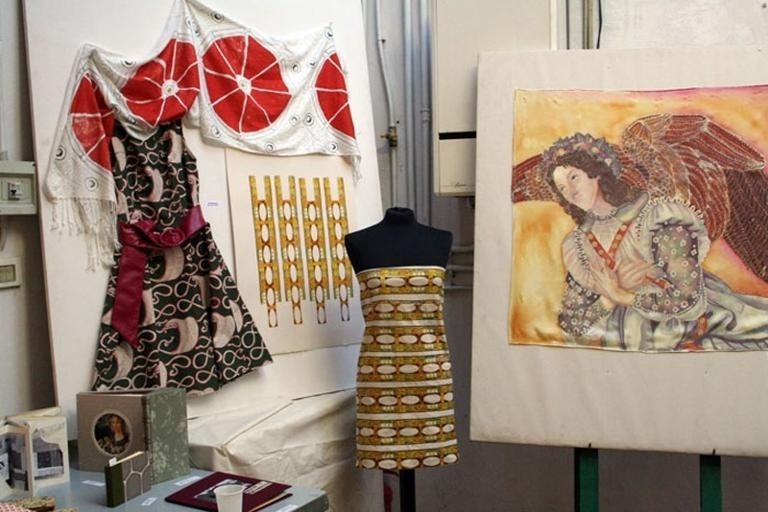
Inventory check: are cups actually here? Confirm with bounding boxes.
[212,484,246,512]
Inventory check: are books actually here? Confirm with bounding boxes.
[163,468,294,512]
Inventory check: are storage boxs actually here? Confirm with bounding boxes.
[74,387,189,485]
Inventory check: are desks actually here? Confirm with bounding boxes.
[0,466,329,512]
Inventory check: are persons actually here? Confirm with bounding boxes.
[339,203,461,473]
[545,145,766,354]
[97,413,131,455]
